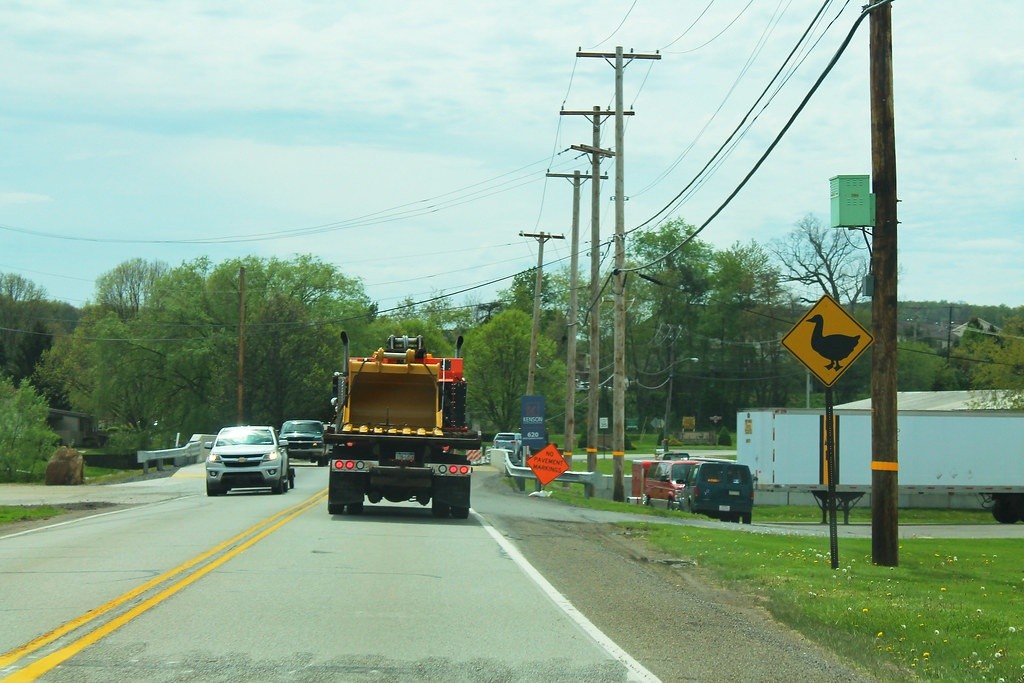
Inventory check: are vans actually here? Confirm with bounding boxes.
[675,461,757,525]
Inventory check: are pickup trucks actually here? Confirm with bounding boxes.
[656,452,690,463]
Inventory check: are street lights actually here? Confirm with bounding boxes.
[663,356,700,452]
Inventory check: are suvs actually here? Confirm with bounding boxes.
[277,420,331,467]
[202,426,296,496]
[493,432,524,452]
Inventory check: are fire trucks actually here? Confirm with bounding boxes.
[631,460,698,508]
[323,328,485,520]
